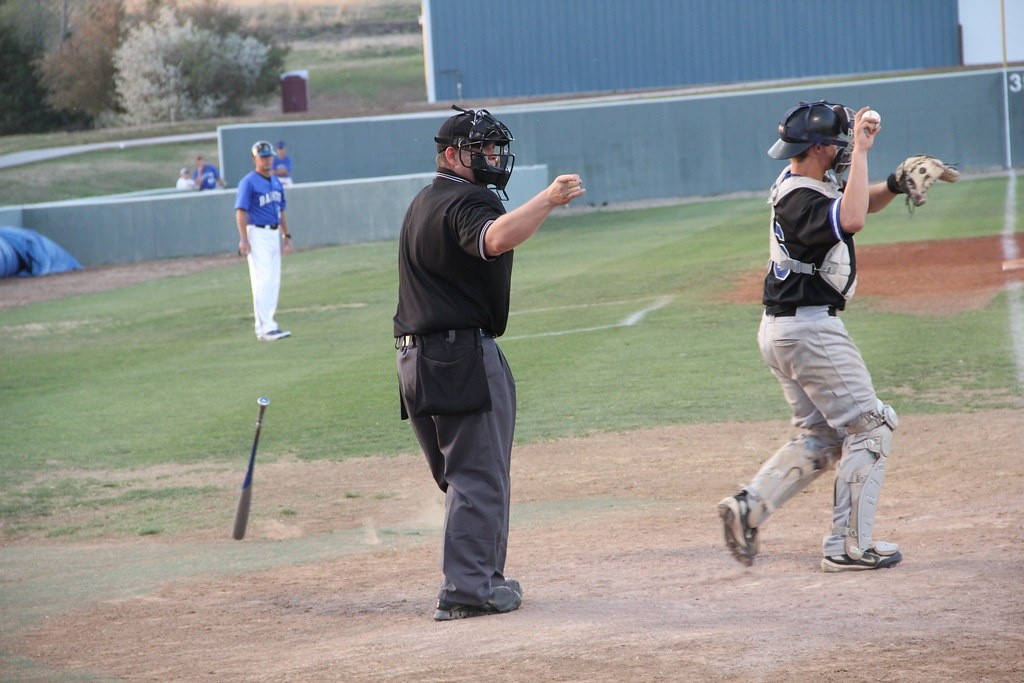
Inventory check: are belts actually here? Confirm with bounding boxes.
[775,306,836,316]
[396,329,487,347]
[256,223,278,229]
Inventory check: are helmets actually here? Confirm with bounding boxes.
[768,103,841,160]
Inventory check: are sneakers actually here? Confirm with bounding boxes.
[821,547,902,573]
[718,490,758,566]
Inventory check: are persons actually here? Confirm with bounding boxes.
[271,140,294,187]
[393,104,586,622]
[176,157,228,191]
[719,99,959,573]
[234,140,294,341]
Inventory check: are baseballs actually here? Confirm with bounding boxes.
[861,109,881,130]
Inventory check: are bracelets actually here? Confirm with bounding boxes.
[239,240,249,243]
[281,233,292,239]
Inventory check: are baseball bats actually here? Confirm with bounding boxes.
[232,397,271,541]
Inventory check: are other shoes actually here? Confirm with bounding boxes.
[434,585,521,620]
[491,578,522,596]
[257,329,290,341]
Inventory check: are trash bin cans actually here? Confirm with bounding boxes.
[279,69,308,113]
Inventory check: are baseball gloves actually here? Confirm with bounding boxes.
[895,154,959,207]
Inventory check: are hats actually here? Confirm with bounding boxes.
[252,141,276,157]
[436,110,509,154]
